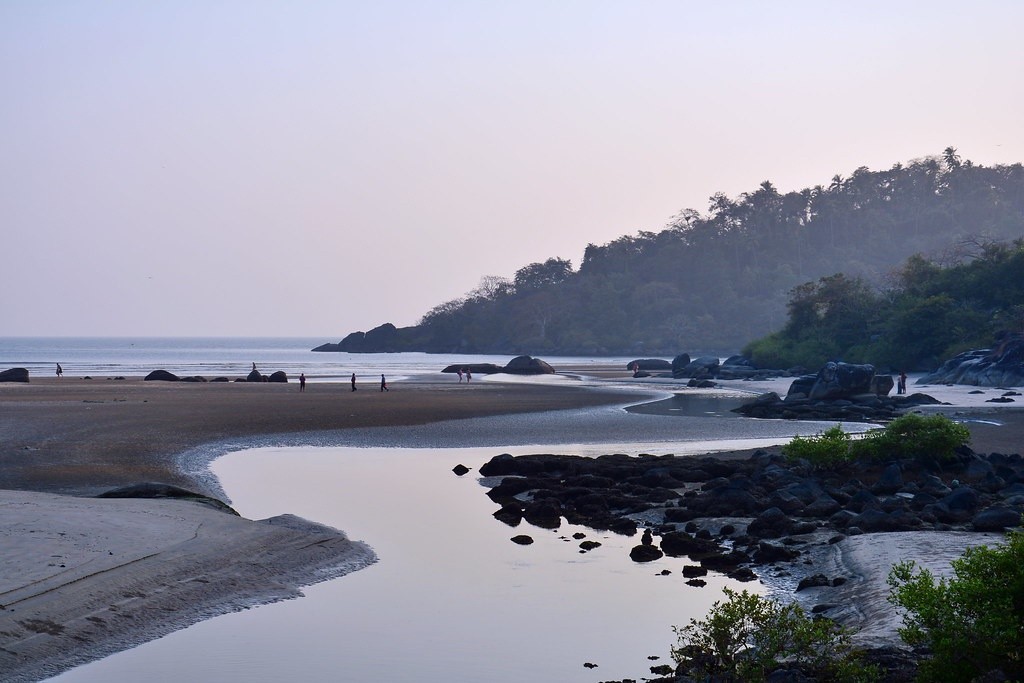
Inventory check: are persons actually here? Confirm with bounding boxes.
[897,373,903,394]
[253,362,256,370]
[458,367,463,384]
[351,373,357,392]
[300,373,306,391]
[466,368,471,384]
[380,374,389,392]
[56,363,62,377]
[902,372,907,394]
